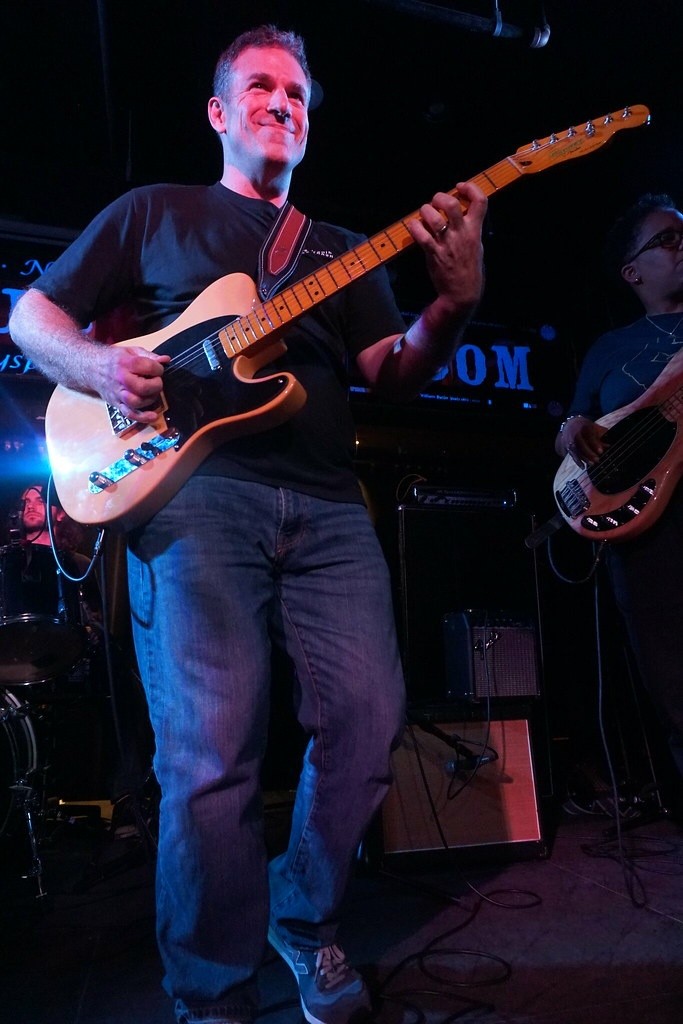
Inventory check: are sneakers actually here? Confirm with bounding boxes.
[267,926,374,1024]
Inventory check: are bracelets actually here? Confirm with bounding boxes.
[560,415,582,433]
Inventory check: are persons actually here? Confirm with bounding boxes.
[9,24,486,1024]
[554,195,683,768]
[0,486,151,840]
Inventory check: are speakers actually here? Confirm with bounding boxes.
[364,494,546,862]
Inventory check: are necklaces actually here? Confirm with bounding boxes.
[646,314,683,335]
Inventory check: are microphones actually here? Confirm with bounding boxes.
[445,754,496,775]
[15,501,27,527]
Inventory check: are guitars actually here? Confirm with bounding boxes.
[551,345,683,541]
[42,99,652,538]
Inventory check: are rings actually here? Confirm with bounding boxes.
[437,224,449,235]
[570,443,576,451]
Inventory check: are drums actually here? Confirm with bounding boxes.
[0,542,91,687]
[0,691,37,840]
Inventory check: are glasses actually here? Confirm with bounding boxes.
[628,226,683,264]
